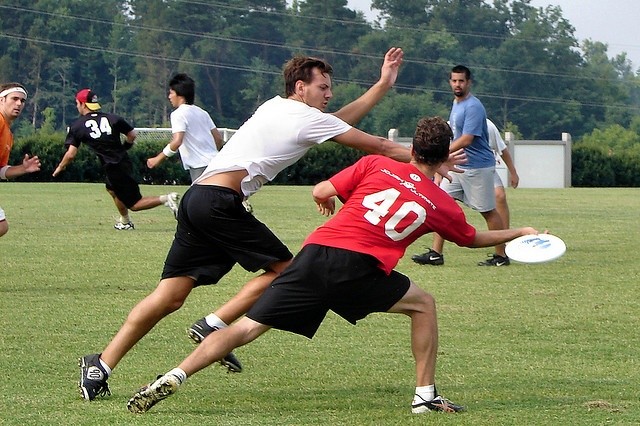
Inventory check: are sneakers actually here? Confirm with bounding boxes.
[77,352,111,402]
[478,253,511,266]
[243,200,254,213]
[127,373,184,414]
[412,244,445,266]
[164,192,181,220]
[114,221,135,230]
[189,316,243,373]
[412,384,464,414]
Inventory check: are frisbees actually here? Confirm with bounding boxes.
[504,233,566,263]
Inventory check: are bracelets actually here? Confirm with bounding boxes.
[162,143,179,158]
[124,141,133,149]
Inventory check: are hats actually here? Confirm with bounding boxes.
[76,89,102,111]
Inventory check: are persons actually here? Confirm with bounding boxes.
[434,119,520,244]
[1,82,42,235]
[78,48,467,402]
[147,74,221,185]
[124,117,550,415]
[411,64,510,267]
[52,89,181,229]
